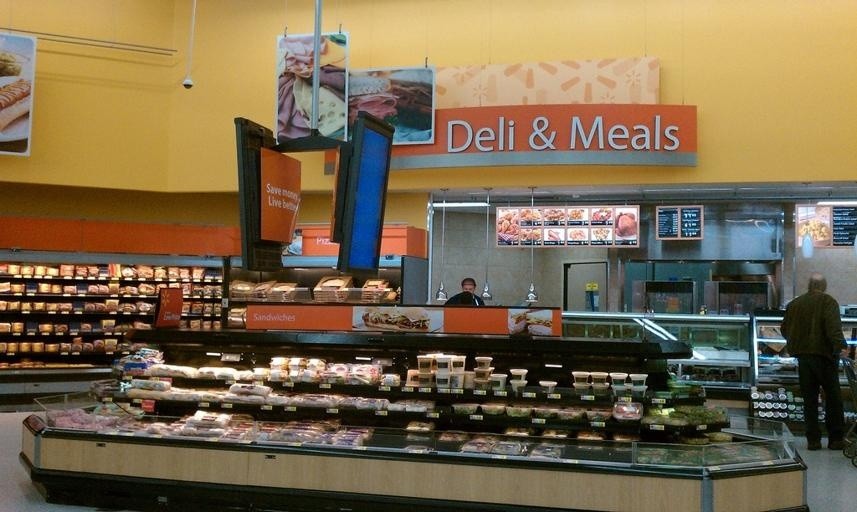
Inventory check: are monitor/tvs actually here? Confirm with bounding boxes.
[330,112,395,279]
[234,117,294,271]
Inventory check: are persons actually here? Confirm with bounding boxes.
[779,272,851,451]
[460,291,474,305]
[443,277,485,307]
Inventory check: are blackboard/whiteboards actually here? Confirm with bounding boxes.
[655,205,704,241]
[832,205,857,248]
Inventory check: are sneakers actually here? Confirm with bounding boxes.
[806,440,850,451]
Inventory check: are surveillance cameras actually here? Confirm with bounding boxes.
[181,79,194,89]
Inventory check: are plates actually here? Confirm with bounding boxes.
[0,74,30,143]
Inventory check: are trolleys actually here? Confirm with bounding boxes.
[841,356,857,468]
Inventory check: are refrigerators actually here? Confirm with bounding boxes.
[748,314,857,390]
